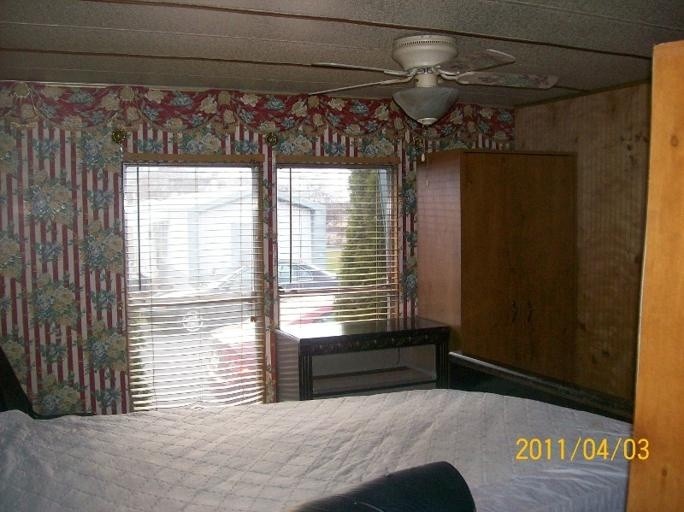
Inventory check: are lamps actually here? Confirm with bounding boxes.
[391,86,459,126]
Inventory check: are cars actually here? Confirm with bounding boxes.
[199,293,338,404]
[146,254,343,334]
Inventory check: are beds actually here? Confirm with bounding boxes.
[0,339,631,512]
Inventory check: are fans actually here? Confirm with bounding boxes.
[301,33,562,102]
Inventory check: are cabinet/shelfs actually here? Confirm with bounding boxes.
[621,37,684,510]
[416,149,578,387]
[276,316,451,401]
